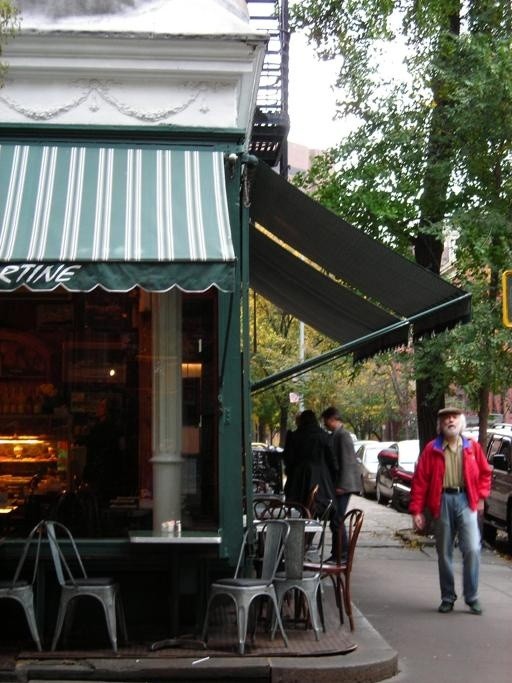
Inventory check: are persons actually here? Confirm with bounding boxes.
[410,406,492,614]
[72,398,123,504]
[284,409,338,546]
[322,406,363,565]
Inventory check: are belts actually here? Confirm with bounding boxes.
[442,485,466,493]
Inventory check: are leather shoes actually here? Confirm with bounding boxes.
[466,596,482,613]
[439,600,453,612]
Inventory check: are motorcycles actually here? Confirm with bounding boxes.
[377,448,415,512]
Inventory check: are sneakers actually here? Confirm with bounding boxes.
[326,551,348,564]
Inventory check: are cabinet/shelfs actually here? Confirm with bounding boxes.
[0,332,140,513]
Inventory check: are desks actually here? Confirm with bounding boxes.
[125,528,222,651]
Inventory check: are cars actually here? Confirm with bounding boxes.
[375,438,420,514]
[353,441,397,497]
[352,439,379,455]
[251,442,284,452]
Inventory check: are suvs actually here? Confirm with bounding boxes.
[477,428,511,549]
[492,423,511,430]
[465,426,479,440]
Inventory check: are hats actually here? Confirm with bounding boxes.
[437,406,461,415]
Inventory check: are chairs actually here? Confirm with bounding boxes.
[252,484,365,643]
[44,520,131,657]
[0,520,42,652]
[202,517,291,657]
